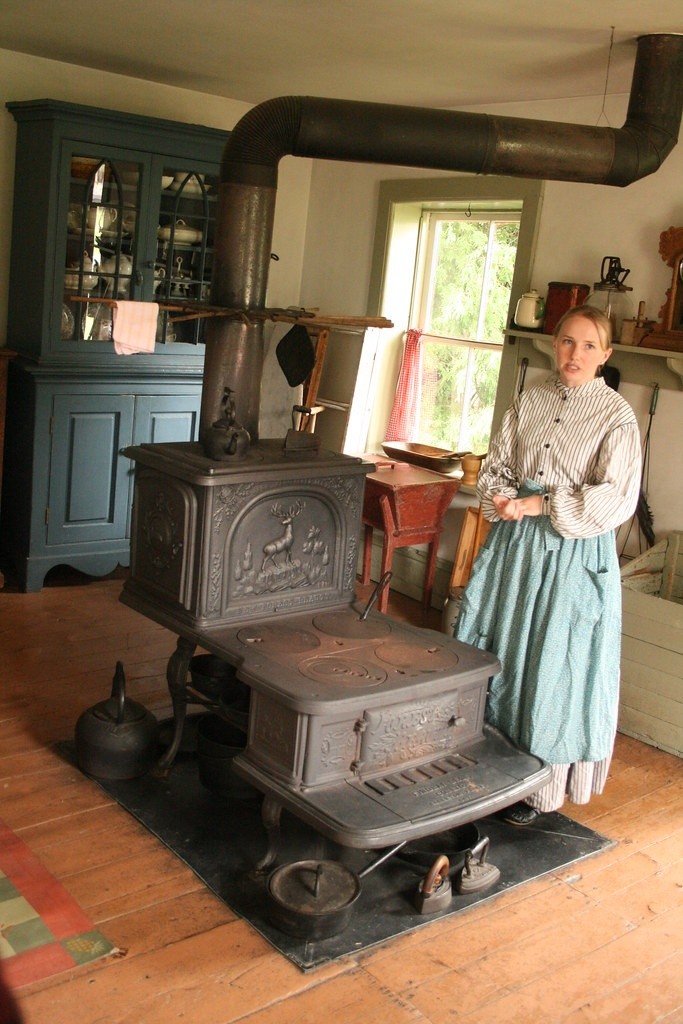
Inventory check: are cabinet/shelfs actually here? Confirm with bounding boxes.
[5,99,233,594]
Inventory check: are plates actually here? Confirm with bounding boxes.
[74,227,129,239]
[169,180,212,195]
[123,170,173,189]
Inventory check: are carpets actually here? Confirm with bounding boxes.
[0,817,119,994]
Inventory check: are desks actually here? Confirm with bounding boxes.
[358,455,461,619]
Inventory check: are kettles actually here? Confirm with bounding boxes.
[203,386,251,460]
[72,660,159,783]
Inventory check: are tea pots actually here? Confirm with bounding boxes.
[65,250,165,295]
[81,206,118,231]
[514,289,545,331]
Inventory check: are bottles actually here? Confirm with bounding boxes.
[583,255,634,344]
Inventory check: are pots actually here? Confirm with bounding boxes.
[264,839,411,941]
[394,820,481,869]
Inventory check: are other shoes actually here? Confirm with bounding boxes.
[504,803,543,825]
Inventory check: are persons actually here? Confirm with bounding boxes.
[452,305,642,823]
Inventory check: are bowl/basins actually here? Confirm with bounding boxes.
[174,171,206,185]
[71,157,101,178]
[157,219,203,245]
